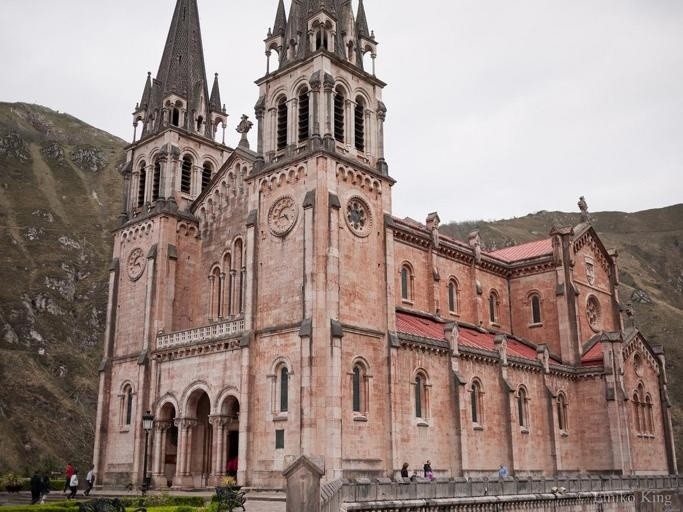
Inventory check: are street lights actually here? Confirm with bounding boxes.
[141,410,154,496]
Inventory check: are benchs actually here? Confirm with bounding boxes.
[215,486,247,512]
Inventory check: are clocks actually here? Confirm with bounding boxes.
[125,245,147,281]
[268,193,299,237]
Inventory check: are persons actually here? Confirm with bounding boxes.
[409,468,419,482]
[400,462,408,478]
[28,468,42,506]
[66,468,80,501]
[423,459,432,478]
[425,468,433,482]
[497,462,508,480]
[39,475,51,506]
[62,462,75,493]
[82,464,96,497]
